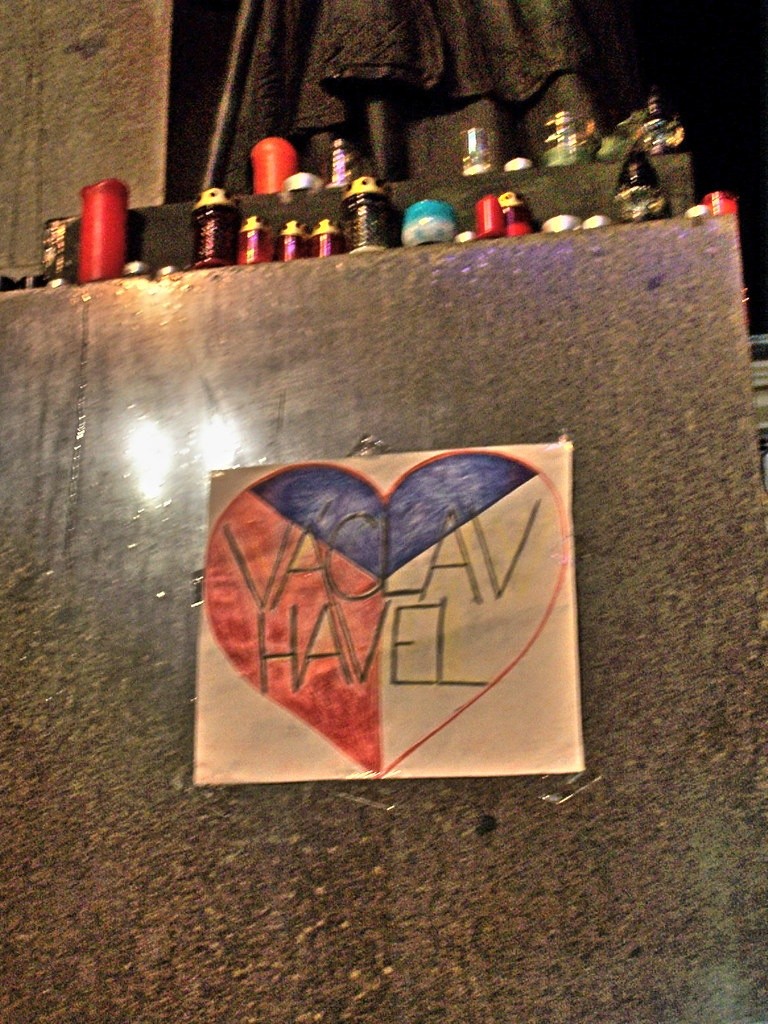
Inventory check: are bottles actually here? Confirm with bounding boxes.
[278,220,305,264]
[498,192,532,236]
[191,189,237,270]
[239,215,273,265]
[340,176,392,254]
[310,219,344,258]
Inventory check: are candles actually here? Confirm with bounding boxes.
[474,194,505,239]
[78,179,129,288]
[251,137,297,195]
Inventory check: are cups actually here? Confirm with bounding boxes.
[251,137,297,195]
[475,194,505,238]
[77,178,130,285]
